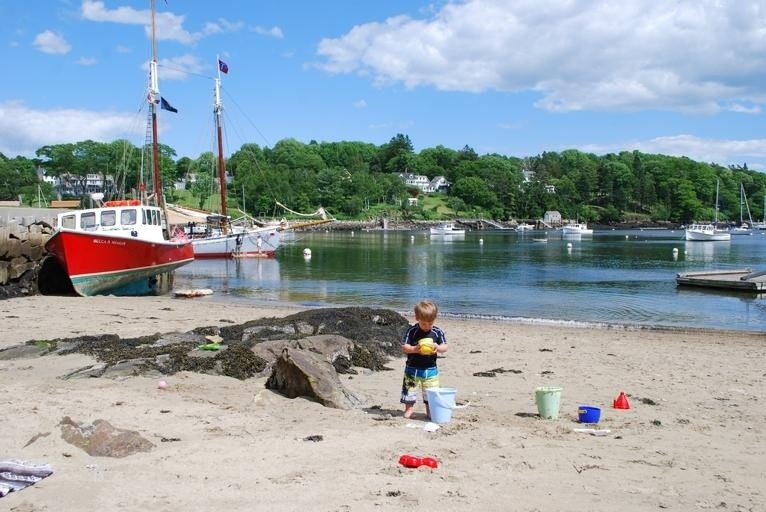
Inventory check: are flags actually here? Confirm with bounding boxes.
[219,60,228,74]
[161,96,177,113]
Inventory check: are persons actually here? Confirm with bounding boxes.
[400,301,448,418]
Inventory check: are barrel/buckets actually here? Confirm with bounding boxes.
[535,386,564,419]
[426,386,470,425]
[573,406,601,423]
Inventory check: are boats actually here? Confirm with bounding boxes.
[563,223,593,233]
[516,222,535,231]
[429,221,465,233]
[683,179,766,241]
